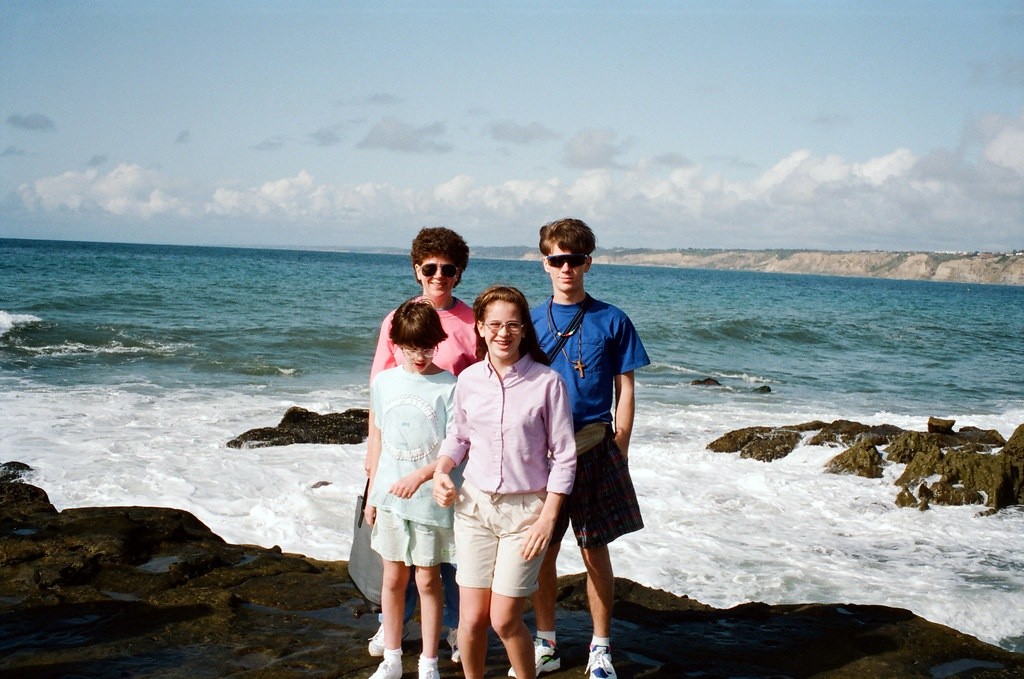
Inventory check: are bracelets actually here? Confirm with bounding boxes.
[432,470,447,474]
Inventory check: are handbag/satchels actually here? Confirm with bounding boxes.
[347,478,384,606]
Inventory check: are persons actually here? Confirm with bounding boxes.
[431,285,577,679]
[364,218,488,679]
[508,220,651,679]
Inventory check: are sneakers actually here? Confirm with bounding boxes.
[418,659,441,679]
[508,638,561,679]
[368,660,403,679]
[367,622,409,656]
[447,627,461,663]
[585,643,617,679]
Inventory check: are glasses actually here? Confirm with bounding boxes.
[420,263,460,277]
[483,320,525,334]
[404,345,439,359]
[545,254,588,268]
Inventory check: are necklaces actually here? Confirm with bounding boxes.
[547,294,588,378]
